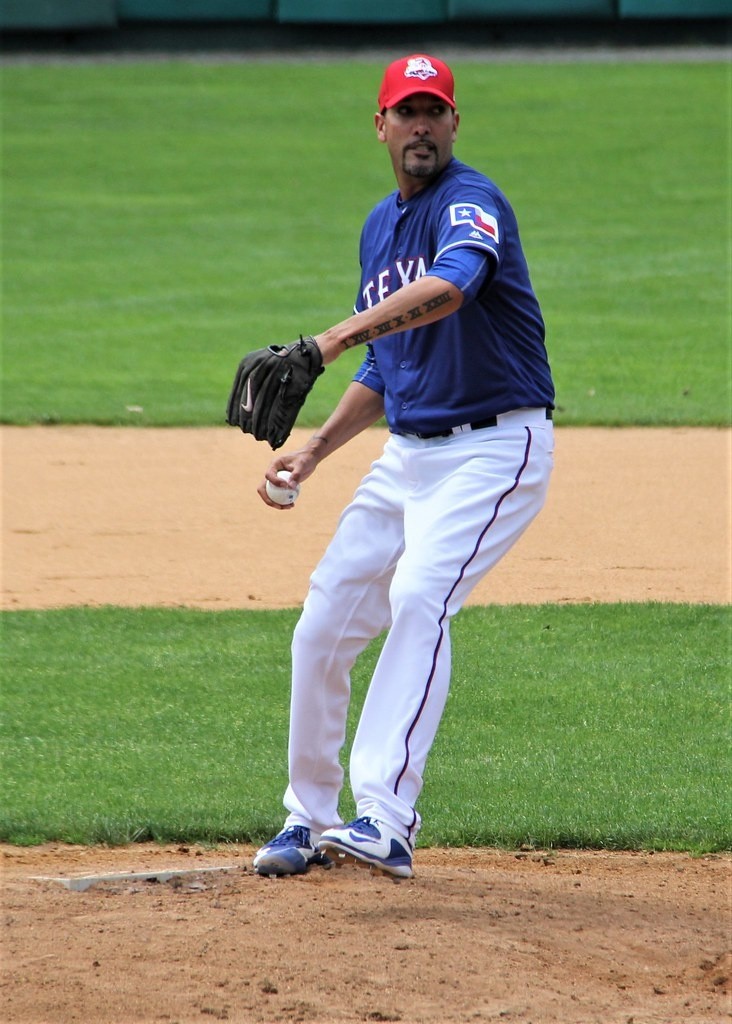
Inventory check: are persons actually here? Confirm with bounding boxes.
[255,54,555,880]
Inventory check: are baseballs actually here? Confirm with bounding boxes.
[266,470,300,504]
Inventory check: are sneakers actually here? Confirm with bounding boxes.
[252,826,330,879]
[317,817,414,878]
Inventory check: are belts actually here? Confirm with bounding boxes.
[406,407,553,438]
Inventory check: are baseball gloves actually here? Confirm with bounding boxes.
[223,334,328,448]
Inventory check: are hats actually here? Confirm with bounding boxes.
[378,53,457,116]
